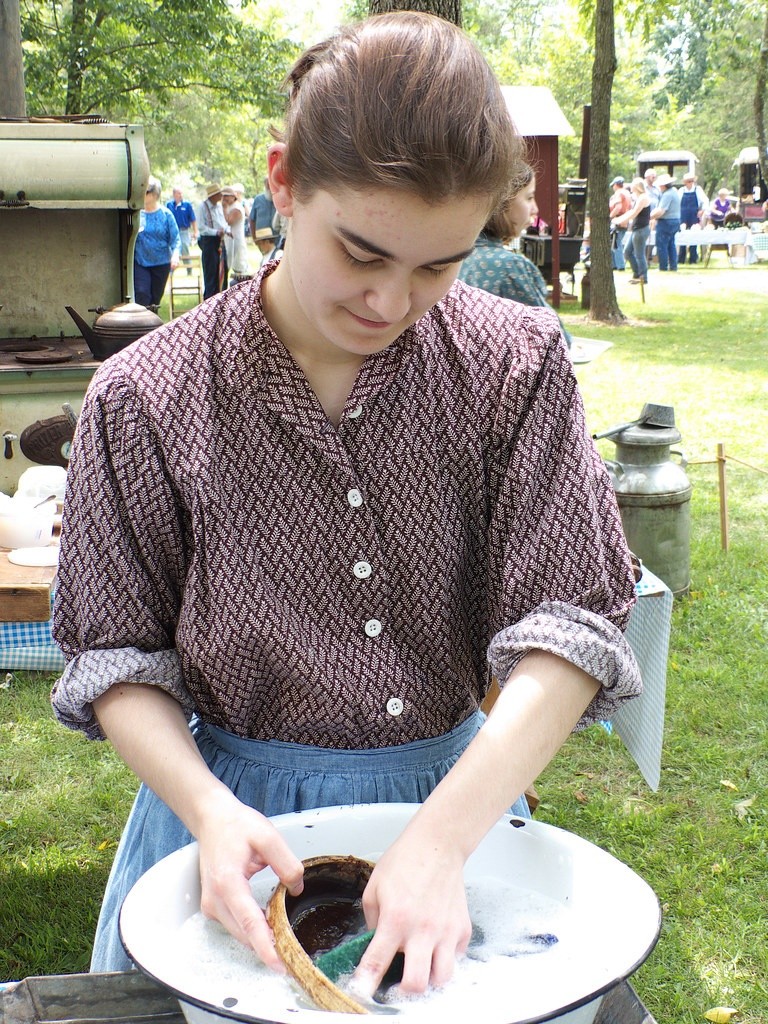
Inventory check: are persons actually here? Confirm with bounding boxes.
[678,172,709,264]
[250,178,290,265]
[134,176,180,313]
[643,169,661,260]
[612,177,651,284]
[650,174,682,272]
[609,176,632,271]
[457,160,571,348]
[167,187,197,275]
[710,188,732,230]
[197,183,232,300]
[234,183,250,218]
[221,186,248,273]
[53,11,644,993]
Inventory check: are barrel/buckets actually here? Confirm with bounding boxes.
[598,423,691,601]
[18,466,67,528]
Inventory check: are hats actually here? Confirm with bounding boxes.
[205,185,222,197]
[610,177,624,187]
[645,168,656,177]
[632,177,645,193]
[254,227,279,241]
[681,172,697,183]
[222,186,235,195]
[652,174,677,186]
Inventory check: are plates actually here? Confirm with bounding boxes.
[8,547,60,567]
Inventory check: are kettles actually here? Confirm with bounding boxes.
[66,296,163,361]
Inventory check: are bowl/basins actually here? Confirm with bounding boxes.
[569,335,613,364]
[264,855,405,1014]
[0,498,59,548]
[117,803,662,1023]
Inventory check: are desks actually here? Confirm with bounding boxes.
[521,235,583,296]
[0,547,61,622]
[0,567,673,797]
[646,226,759,268]
[752,235,768,251]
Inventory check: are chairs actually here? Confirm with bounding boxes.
[170,255,201,321]
